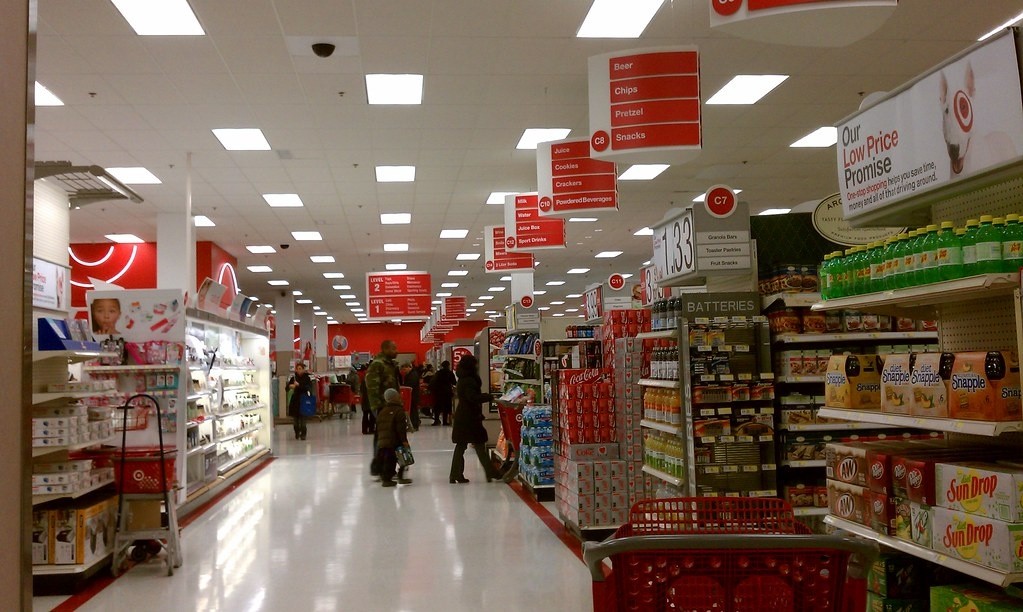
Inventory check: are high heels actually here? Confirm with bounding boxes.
[486,475,504,483]
[450,474,469,483]
[382,480,397,487]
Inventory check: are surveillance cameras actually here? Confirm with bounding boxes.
[311,43,335,57]
[280,244,289,249]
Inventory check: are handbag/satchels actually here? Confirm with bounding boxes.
[299,386,316,416]
[395,446,414,467]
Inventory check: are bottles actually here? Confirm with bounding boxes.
[650,297,686,331]
[644,388,681,427]
[645,434,683,478]
[818,215,1023,300]
[650,347,678,381]
[518,406,555,485]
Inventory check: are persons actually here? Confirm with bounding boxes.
[285,364,312,439]
[360,358,376,434]
[196,279,212,310]
[89,514,115,553]
[302,342,312,366]
[450,355,502,483]
[338,344,343,350]
[264,309,271,329]
[347,367,358,414]
[393,359,457,431]
[235,332,241,355]
[365,339,402,475]
[92,299,121,335]
[377,389,411,487]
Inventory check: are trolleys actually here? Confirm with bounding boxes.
[400,386,415,433]
[416,383,436,424]
[108,394,183,577]
[582,497,879,612]
[326,383,360,420]
[491,399,526,484]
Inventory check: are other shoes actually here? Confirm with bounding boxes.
[432,421,440,426]
[443,423,450,426]
[363,430,377,434]
[296,432,299,439]
[300,436,306,440]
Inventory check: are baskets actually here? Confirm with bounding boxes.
[112,449,180,495]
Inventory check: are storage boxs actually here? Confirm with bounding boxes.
[770,309,1023,612]
[550,309,651,528]
[32,405,120,564]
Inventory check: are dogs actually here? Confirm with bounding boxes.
[936,59,1015,180]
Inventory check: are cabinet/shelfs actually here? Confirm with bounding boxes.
[811,273,1023,588]
[504,328,542,406]
[542,338,595,377]
[31,351,116,574]
[82,366,188,503]
[762,292,940,515]
[636,331,683,529]
[187,366,268,498]
